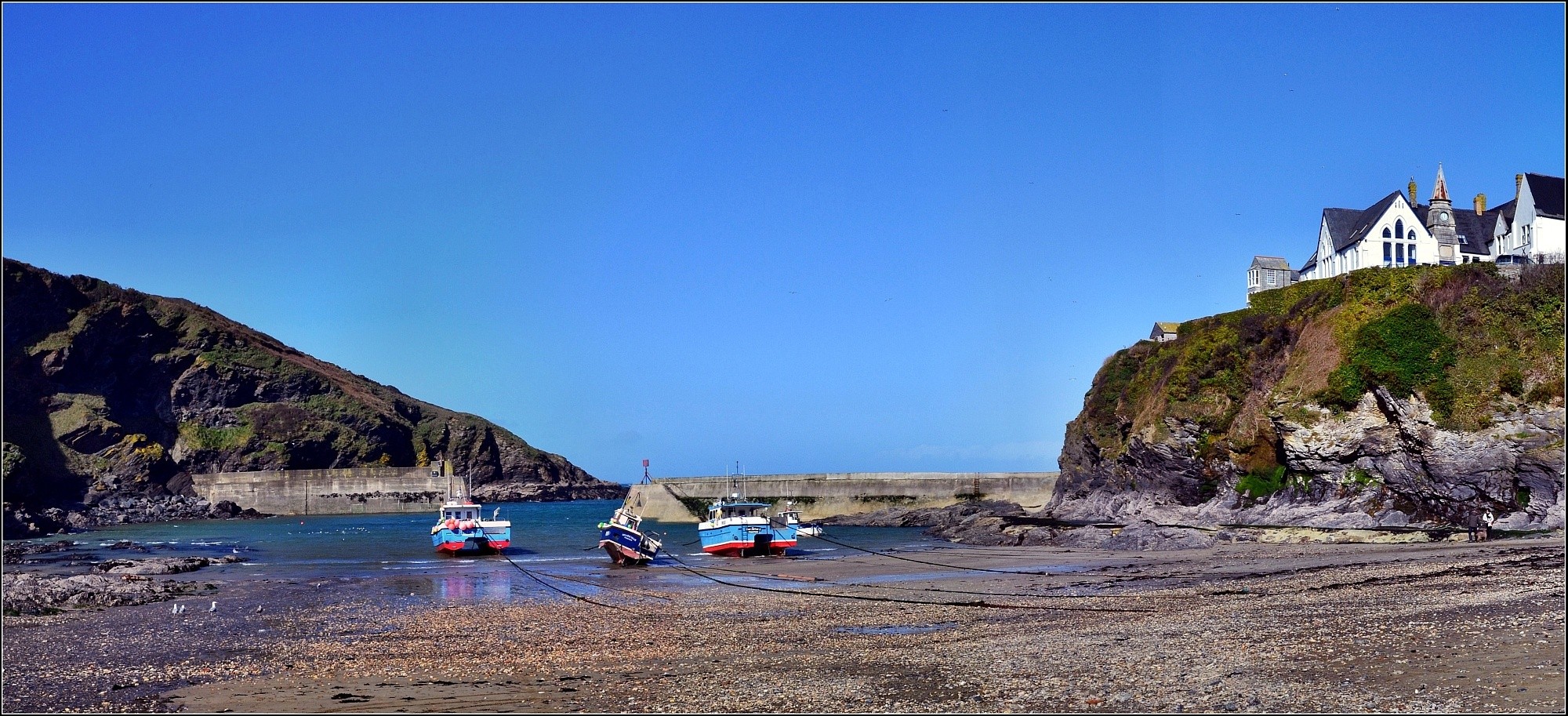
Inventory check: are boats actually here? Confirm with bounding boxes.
[698,465,797,557]
[431,489,510,556]
[777,503,823,537]
[597,487,663,565]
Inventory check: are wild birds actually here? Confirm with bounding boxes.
[171,601,263,616]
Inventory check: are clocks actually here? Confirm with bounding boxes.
[1440,212,1449,222]
